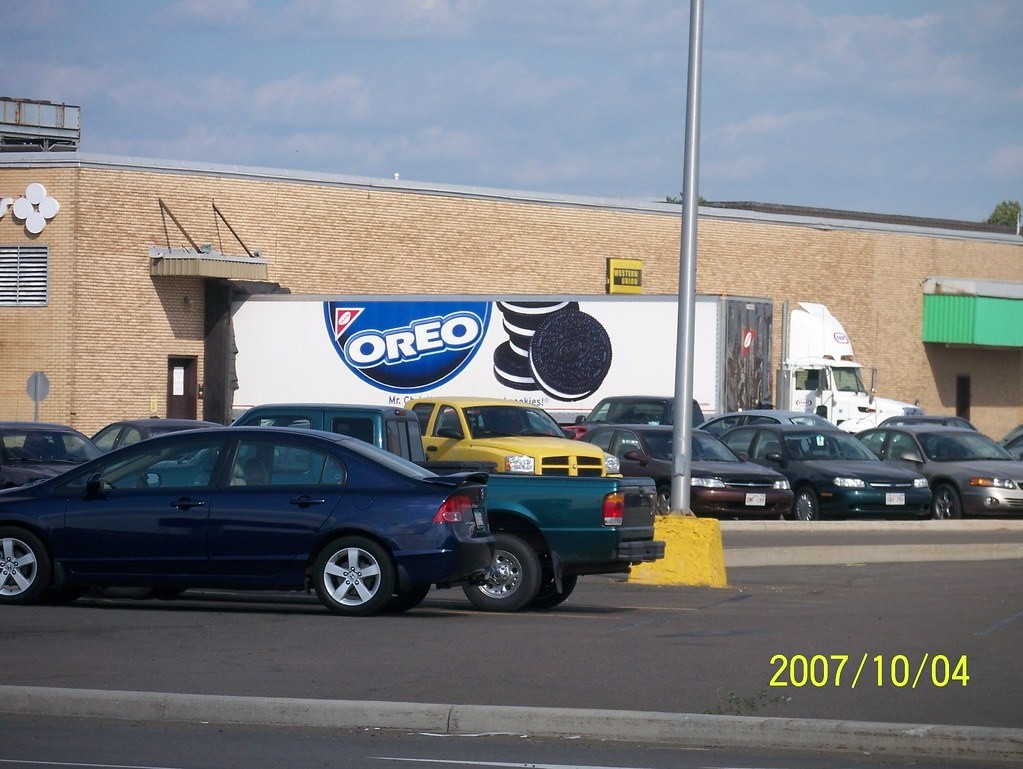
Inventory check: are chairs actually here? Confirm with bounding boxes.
[23,433,49,458]
[230,458,267,486]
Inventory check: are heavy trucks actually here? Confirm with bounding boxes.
[230,293,923,447]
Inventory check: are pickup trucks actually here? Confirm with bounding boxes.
[115,402,667,612]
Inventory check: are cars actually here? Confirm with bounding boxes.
[853,427,1023,520]
[0,420,105,491]
[92,417,224,454]
[0,425,495,616]
[575,426,793,521]
[720,424,932,521]
[692,410,840,438]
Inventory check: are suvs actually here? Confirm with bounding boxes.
[557,395,703,441]
[405,398,622,479]
